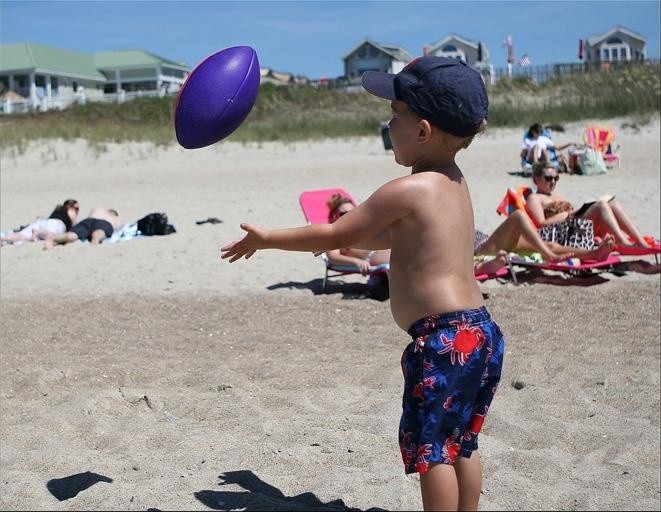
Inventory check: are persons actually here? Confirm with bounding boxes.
[218,54,504,512]
[1,200,81,247]
[44,207,122,251]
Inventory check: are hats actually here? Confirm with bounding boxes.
[359,53,490,140]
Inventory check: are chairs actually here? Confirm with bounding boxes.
[584,125,621,169]
[509,250,623,275]
[520,125,563,176]
[298,188,520,295]
[495,189,659,267]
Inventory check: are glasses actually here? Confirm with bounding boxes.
[540,173,562,184]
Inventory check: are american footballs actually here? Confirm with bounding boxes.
[173,44,259,149]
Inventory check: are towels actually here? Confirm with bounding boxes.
[0,220,141,244]
